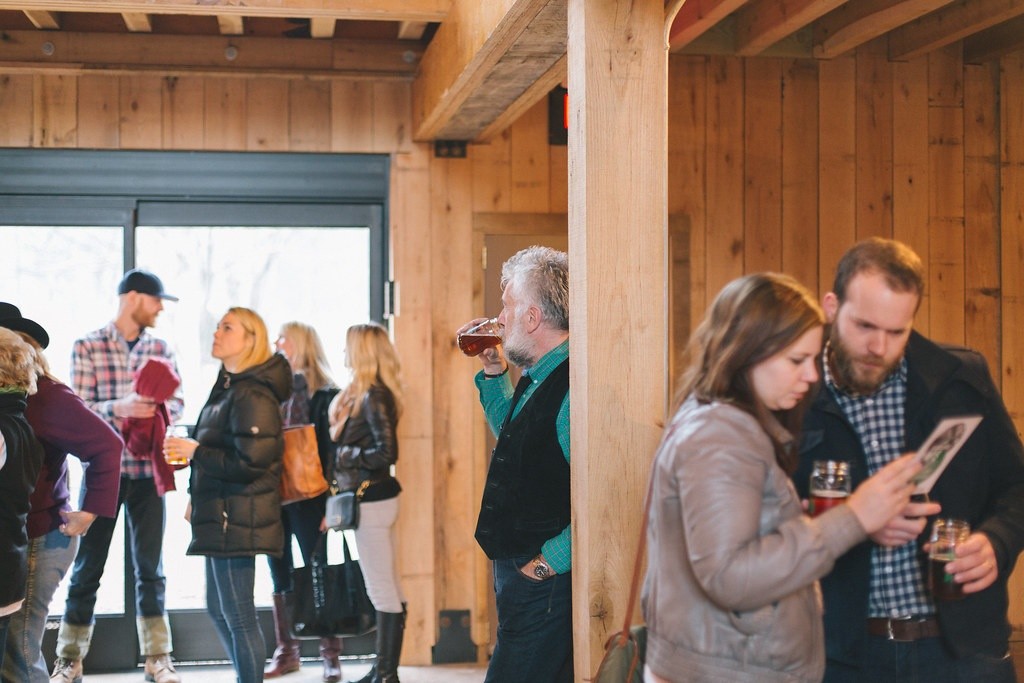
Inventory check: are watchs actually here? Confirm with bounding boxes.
[533,553,551,580]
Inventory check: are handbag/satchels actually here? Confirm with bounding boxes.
[590,624,649,683]
[280,529,376,639]
[324,491,359,531]
[280,423,329,506]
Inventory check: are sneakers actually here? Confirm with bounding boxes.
[49,658,83,682]
[145,653,181,683]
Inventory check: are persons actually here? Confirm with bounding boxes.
[791,236,1024,682]
[50,268,185,683]
[641,272,921,682]
[162,304,292,683]
[0,301,124,683]
[454,244,575,683]
[328,320,407,682]
[264,321,341,683]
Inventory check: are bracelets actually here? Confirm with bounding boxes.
[484,362,509,379]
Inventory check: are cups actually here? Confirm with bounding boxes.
[457,317,506,358]
[165,426,188,466]
[928,519,970,601]
[810,460,853,522]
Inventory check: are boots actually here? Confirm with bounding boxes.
[262,590,301,677]
[320,634,342,682]
[348,601,406,683]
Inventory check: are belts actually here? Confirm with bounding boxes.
[866,614,940,641]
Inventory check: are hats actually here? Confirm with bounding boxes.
[118,270,178,302]
[0,302,49,350]
